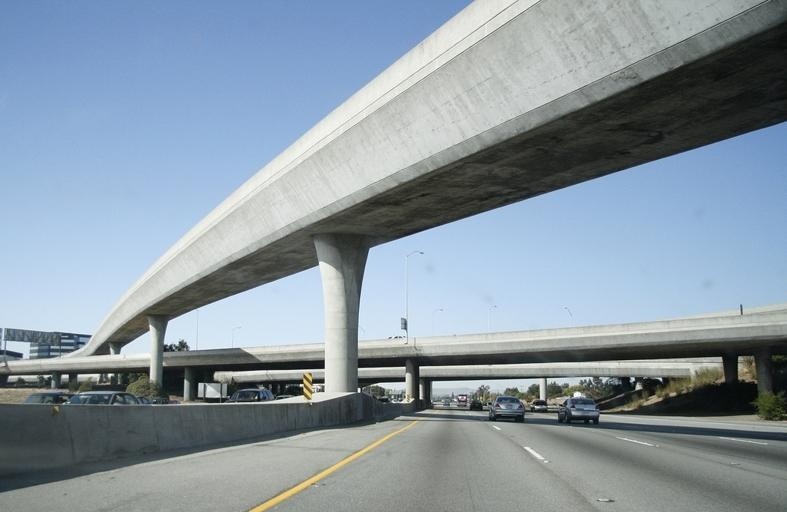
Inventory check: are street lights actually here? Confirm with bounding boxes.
[486,301,498,336]
[560,304,575,327]
[403,248,426,338]
[230,325,242,347]
[430,305,444,339]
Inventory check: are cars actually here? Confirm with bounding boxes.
[487,396,525,423]
[556,396,599,425]
[386,334,404,339]
[23,384,297,406]
[441,393,548,413]
